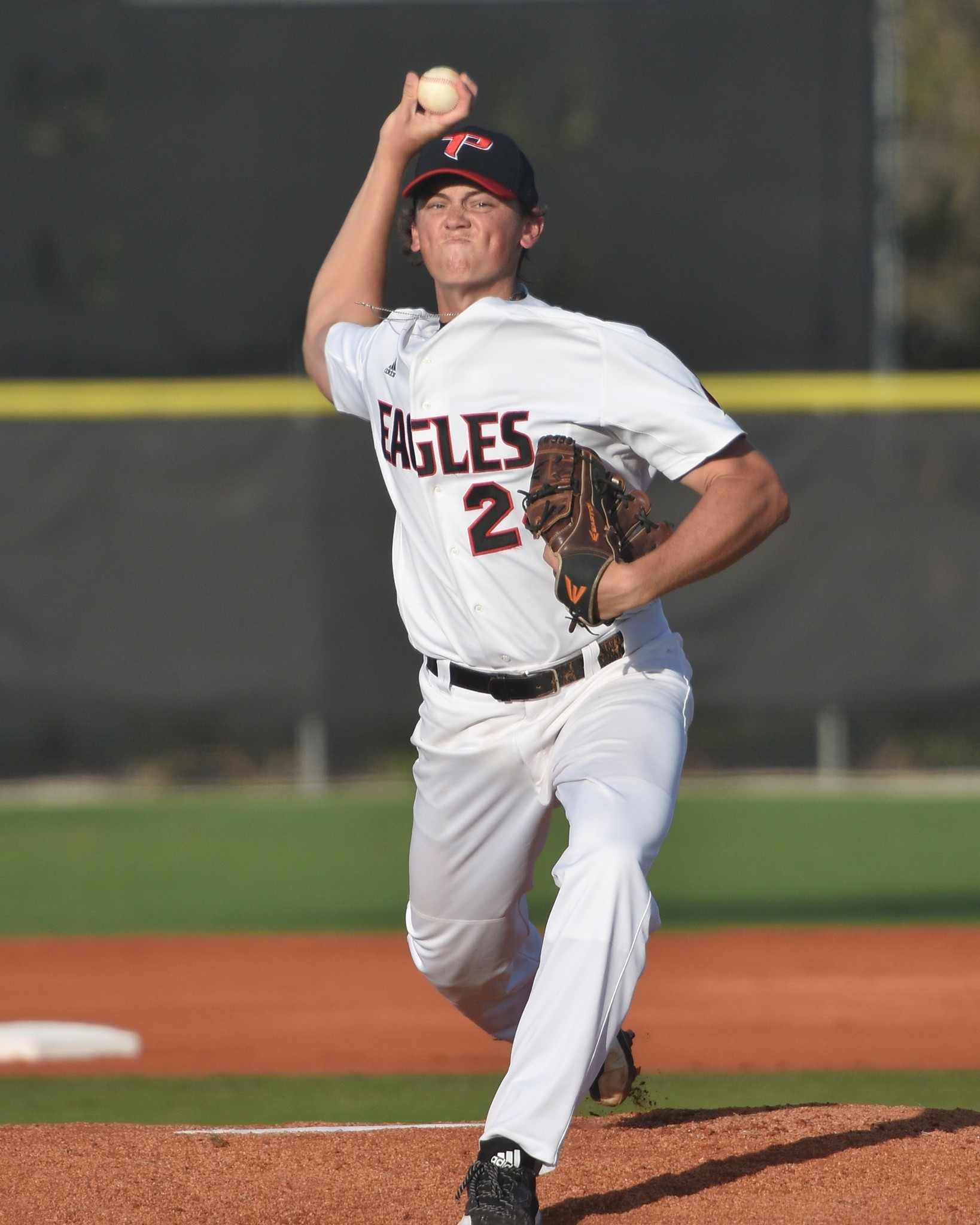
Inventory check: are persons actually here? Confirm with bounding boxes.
[300,73,793,1225]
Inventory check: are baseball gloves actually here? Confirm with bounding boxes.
[523,432,673,628]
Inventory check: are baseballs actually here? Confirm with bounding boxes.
[416,66,464,115]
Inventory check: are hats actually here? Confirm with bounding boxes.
[400,124,539,213]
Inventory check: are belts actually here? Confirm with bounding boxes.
[425,630,628,704]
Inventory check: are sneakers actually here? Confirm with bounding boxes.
[452,1146,541,1225]
[585,1027,641,1108]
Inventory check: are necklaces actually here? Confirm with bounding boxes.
[354,290,526,323]
